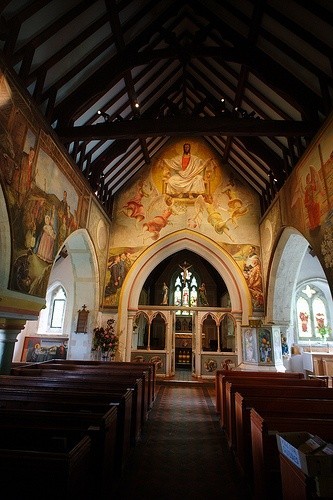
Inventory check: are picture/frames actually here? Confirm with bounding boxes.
[18,335,70,363]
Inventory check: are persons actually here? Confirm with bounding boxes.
[179,264,192,284]
[162,282,169,304]
[199,283,208,306]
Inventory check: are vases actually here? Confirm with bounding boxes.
[100,350,115,362]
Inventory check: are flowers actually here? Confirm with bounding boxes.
[91,327,126,357]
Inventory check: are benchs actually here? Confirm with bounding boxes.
[216,371,333,500]
[0,359,156,500]
